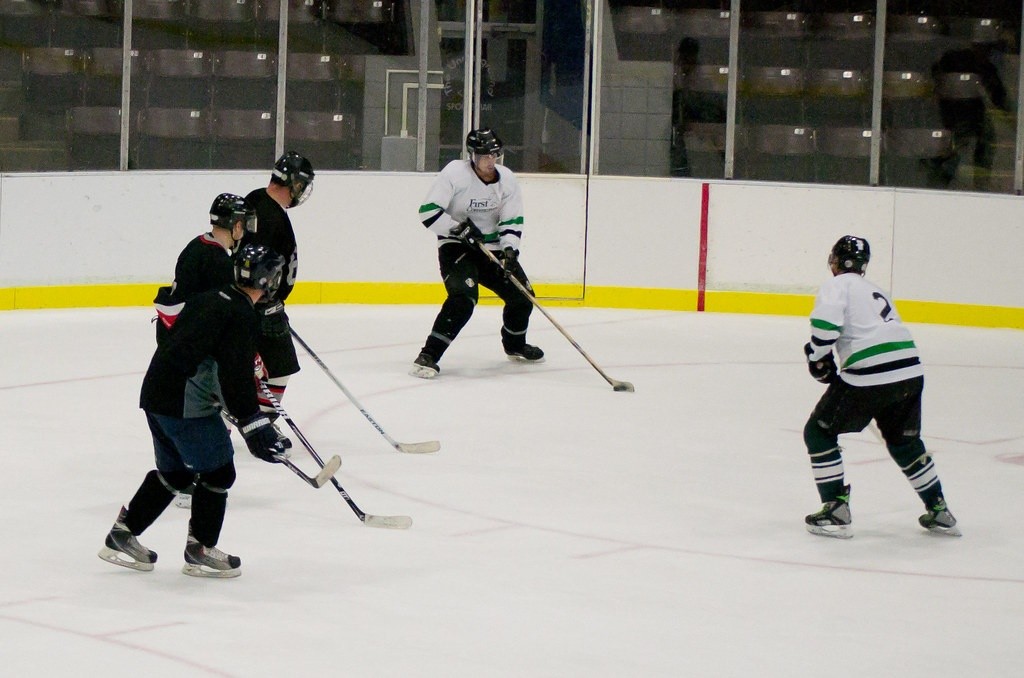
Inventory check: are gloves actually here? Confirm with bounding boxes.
[254,354,269,383]
[237,409,292,463]
[451,217,486,253]
[804,342,838,385]
[498,246,521,286]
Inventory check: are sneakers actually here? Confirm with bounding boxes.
[262,411,292,449]
[409,346,439,379]
[805,484,854,539]
[175,473,201,509]
[501,326,545,363]
[182,518,241,578]
[919,495,962,537]
[96,505,158,571]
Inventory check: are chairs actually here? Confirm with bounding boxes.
[0,1,1002,192]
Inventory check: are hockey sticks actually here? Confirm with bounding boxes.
[218,405,341,489]
[465,232,636,394]
[286,321,441,456]
[256,378,414,530]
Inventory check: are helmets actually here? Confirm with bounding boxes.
[832,235,870,276]
[233,243,285,303]
[209,192,258,234]
[272,150,316,209]
[466,127,504,155]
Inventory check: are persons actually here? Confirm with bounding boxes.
[104,149,315,570]
[408,128,544,374]
[803,235,957,529]
